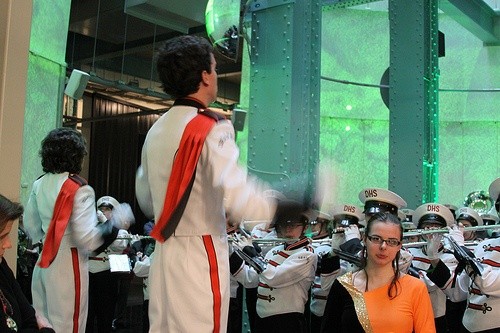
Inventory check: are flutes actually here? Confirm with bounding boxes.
[108,210,500,248]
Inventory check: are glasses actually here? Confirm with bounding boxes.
[366,234,402,247]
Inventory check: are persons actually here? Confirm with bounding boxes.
[226,177,500,333]
[135,36,337,333]
[85,196,155,333]
[0,194,43,333]
[22,127,134,332]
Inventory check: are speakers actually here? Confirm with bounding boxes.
[64,69,90,101]
[231,109,247,131]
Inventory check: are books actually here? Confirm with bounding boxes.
[109,254,131,273]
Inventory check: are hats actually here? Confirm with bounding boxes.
[97,196,120,210]
[358,187,407,215]
[274,210,311,225]
[403,222,417,233]
[312,210,334,225]
[479,214,499,225]
[400,209,414,222]
[454,207,483,227]
[261,189,287,201]
[329,202,365,226]
[412,202,455,228]
[489,178,500,211]
